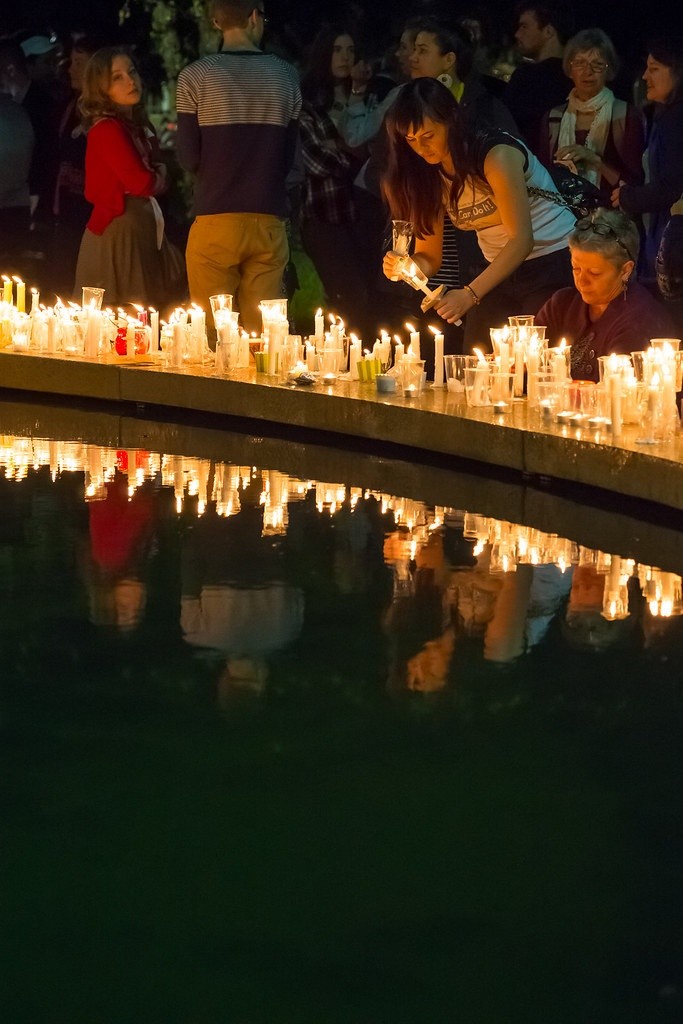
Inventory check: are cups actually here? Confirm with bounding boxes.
[0,433,682,620]
[0,285,683,448]
[392,253,429,291]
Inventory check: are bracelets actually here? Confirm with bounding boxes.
[352,88,367,96]
[465,285,480,306]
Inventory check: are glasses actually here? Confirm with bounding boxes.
[249,7,269,26]
[569,58,609,73]
[574,219,635,266]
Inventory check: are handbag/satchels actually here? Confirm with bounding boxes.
[282,262,300,306]
[549,168,607,219]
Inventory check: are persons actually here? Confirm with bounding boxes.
[297,1,682,382]
[179,500,320,726]
[379,77,578,395]
[176,0,302,363]
[489,206,683,421]
[331,500,682,726]
[77,468,164,633]
[71,47,171,351]
[0,29,108,298]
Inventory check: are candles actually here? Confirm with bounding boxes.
[1,223,682,435]
[1,439,678,619]
